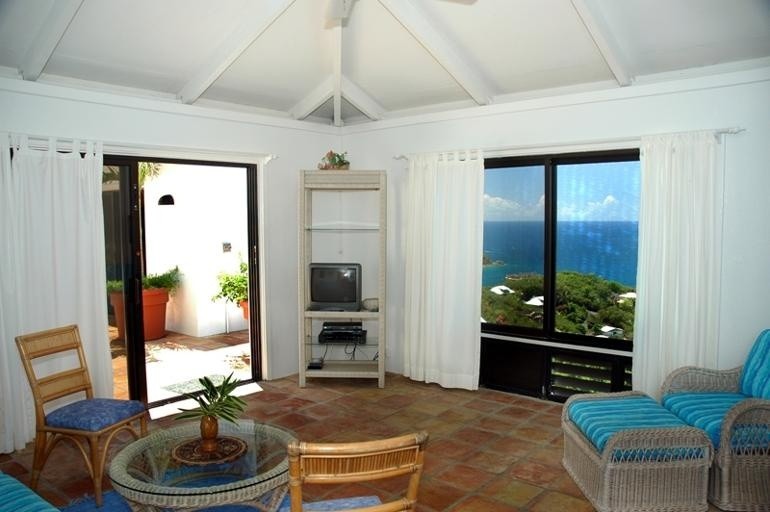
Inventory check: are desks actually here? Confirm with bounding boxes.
[109,420,299,512]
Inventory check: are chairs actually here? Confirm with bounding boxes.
[14,324,150,507]
[663,328,769,509]
[286,429,430,510]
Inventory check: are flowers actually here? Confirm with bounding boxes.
[318,149,350,169]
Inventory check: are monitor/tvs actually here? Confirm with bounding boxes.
[307,263,361,311]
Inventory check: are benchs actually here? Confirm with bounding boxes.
[559,390,714,512]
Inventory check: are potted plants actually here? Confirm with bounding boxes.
[105,265,180,340]
[171,368,249,453]
[211,259,249,321]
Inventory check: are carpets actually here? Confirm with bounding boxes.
[64,462,292,512]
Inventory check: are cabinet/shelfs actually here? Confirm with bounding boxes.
[299,167,389,388]
